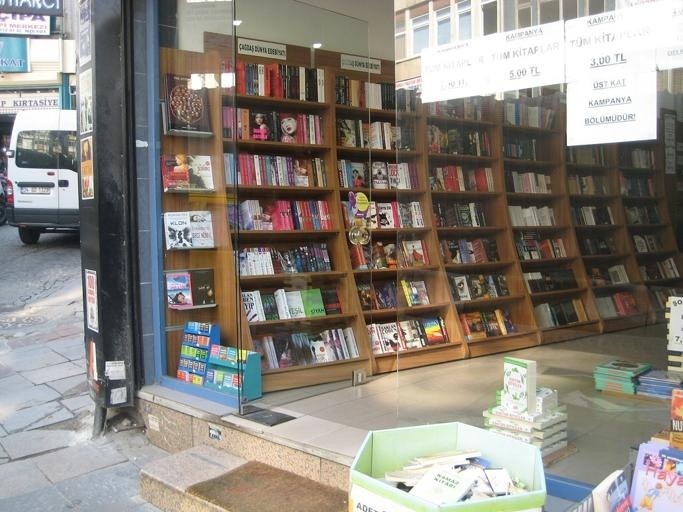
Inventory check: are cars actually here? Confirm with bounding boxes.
[0,159,8,225]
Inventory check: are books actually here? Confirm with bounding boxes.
[593,358,682,399]
[566,144,680,318]
[241,269,579,323]
[161,153,214,190]
[571,389,682,512]
[385,452,527,505]
[221,105,538,159]
[483,357,577,467]
[255,299,588,369]
[236,230,567,276]
[163,73,211,132]
[227,190,556,230]
[236,61,557,128]
[666,296,683,370]
[224,153,552,194]
[163,268,216,306]
[165,211,215,250]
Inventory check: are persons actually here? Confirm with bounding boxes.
[79,140,92,161]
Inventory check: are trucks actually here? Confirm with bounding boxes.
[3,105,80,244]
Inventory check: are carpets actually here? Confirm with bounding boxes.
[188,460,348,512]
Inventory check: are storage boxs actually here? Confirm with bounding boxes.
[349,420,547,512]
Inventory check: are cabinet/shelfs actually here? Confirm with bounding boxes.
[159,30,467,397]
[424,84,683,358]
